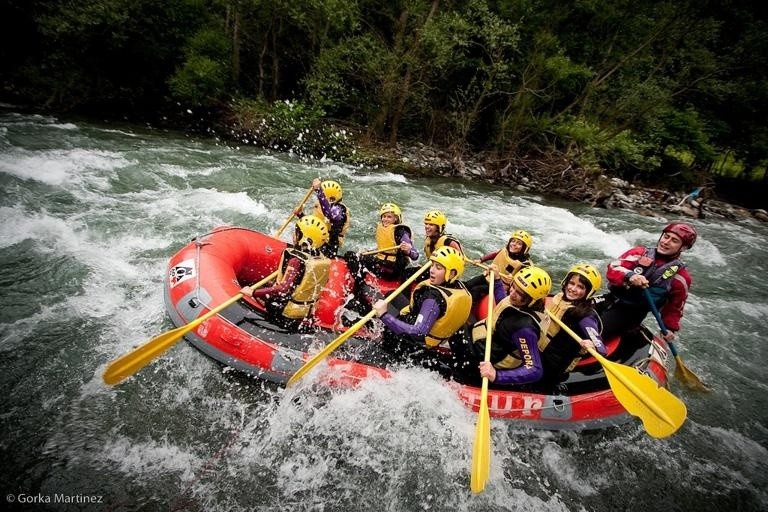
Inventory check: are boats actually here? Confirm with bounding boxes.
[164,226,669,431]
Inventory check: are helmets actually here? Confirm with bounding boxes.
[508,230,532,255]
[294,217,328,251]
[424,211,446,234]
[561,264,601,302]
[380,203,403,225]
[510,266,551,308]
[429,246,465,284]
[657,222,697,257]
[320,181,343,205]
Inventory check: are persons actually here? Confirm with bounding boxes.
[356,246,472,365]
[400,211,463,301]
[532,264,602,396]
[345,203,418,308]
[593,223,696,342]
[441,267,553,388]
[239,217,333,331]
[294,181,350,259]
[463,230,532,305]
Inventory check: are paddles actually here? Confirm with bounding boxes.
[544,306,687,437]
[285,260,434,387]
[103,270,279,384]
[641,281,710,394]
[471,269,495,494]
